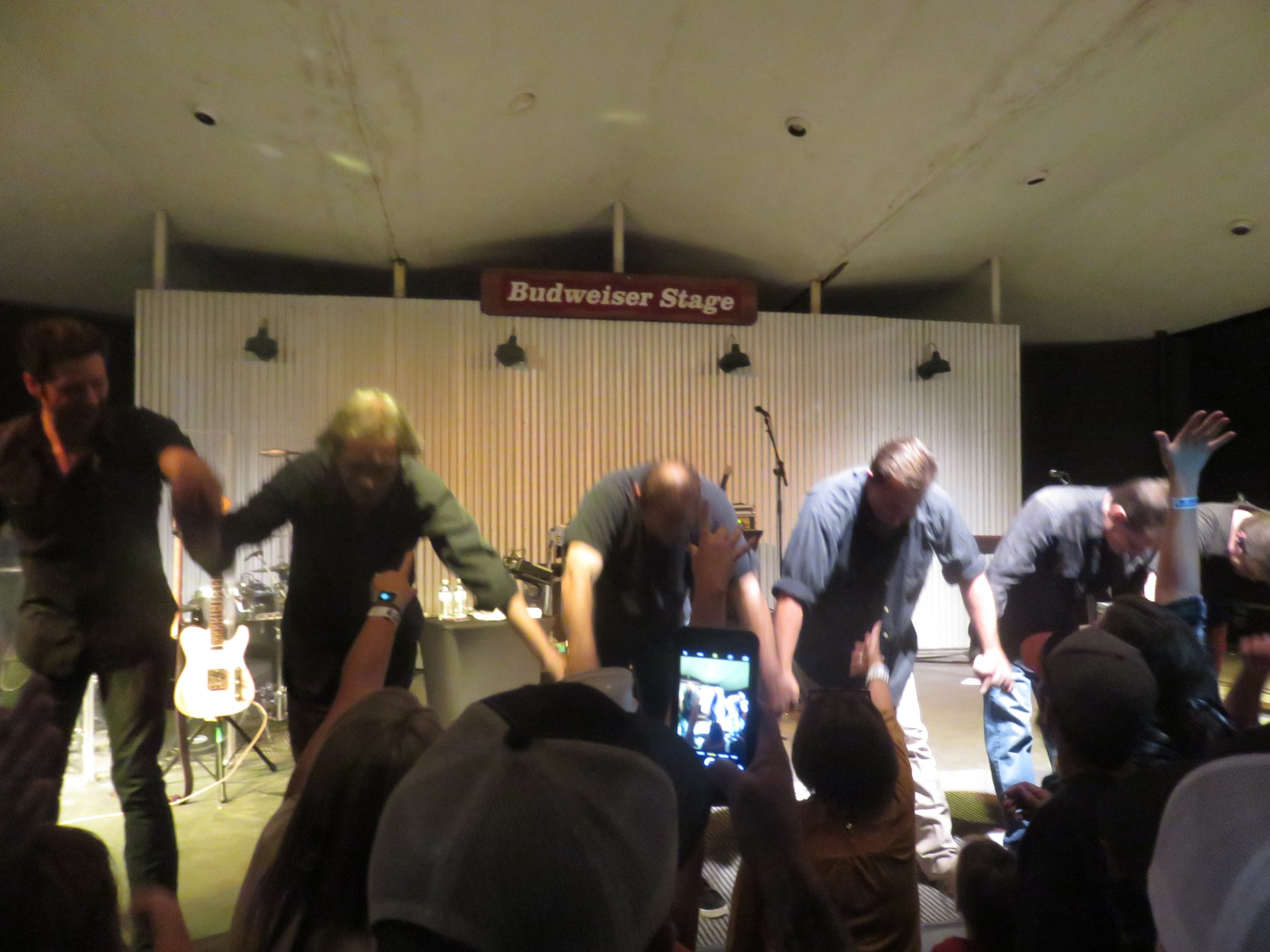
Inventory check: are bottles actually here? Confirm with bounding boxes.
[449,578,468,619]
[438,579,452,622]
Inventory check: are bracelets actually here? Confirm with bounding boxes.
[862,660,890,691]
[1165,494,1197,511]
[366,605,402,625]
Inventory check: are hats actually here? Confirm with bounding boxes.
[1018,623,1161,757]
[365,666,714,952]
[1148,751,1270,951]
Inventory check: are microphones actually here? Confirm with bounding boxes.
[754,405,770,417]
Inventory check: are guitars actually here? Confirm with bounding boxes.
[173,496,255,722]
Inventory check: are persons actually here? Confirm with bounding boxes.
[0,315,1270,952]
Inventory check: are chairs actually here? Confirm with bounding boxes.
[1146,754,1269,952]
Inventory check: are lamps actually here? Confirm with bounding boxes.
[243,319,278,361]
[720,337,751,371]
[918,341,952,382]
[494,335,528,366]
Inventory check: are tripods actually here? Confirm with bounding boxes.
[160,594,287,806]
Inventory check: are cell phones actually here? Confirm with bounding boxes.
[672,625,759,776]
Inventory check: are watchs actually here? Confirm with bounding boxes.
[369,591,405,614]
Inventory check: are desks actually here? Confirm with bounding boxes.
[419,611,540,726]
